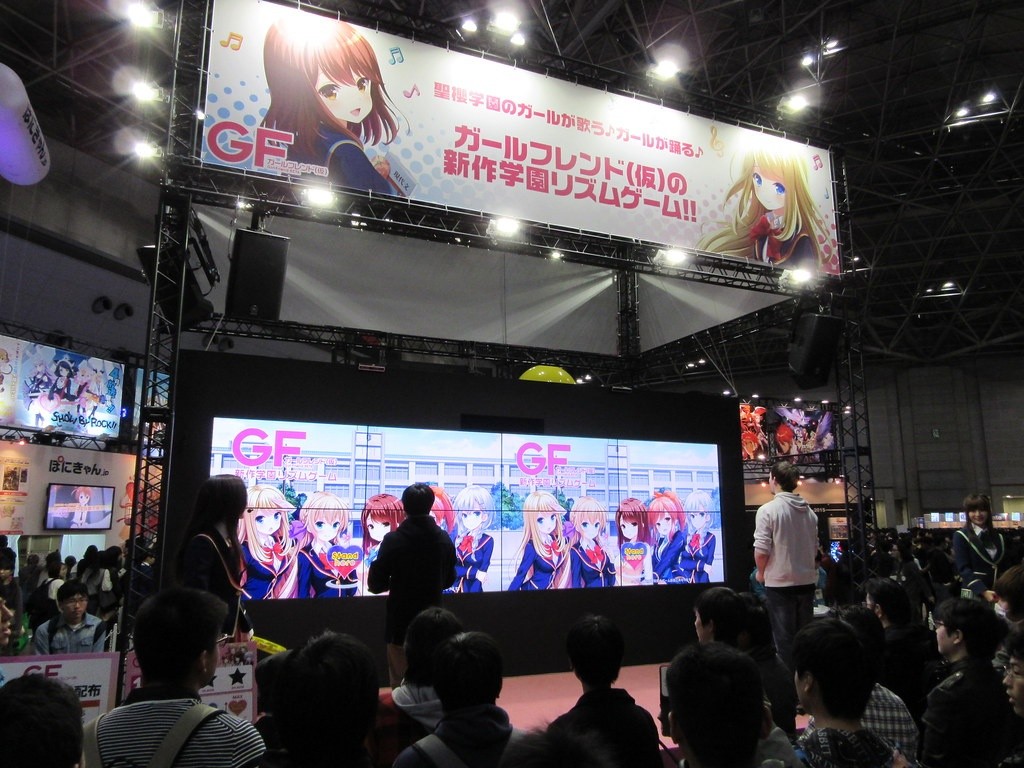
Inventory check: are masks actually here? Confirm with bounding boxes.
[994,603,1023,634]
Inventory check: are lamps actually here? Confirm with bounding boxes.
[203,334,220,348]
[94,297,112,312]
[219,338,236,351]
[115,304,134,320]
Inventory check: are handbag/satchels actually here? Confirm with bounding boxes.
[96,569,117,612]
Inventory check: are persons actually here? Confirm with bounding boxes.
[365,608,462,768]
[252,636,380,768]
[547,615,659,768]
[392,632,528,768]
[952,493,1009,607]
[0,673,85,768]
[694,528,1024,768]
[753,461,818,671]
[367,483,458,686]
[174,474,254,645]
[81,587,266,768]
[657,641,802,768]
[0,536,151,657]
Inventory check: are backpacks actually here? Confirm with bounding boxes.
[27,578,59,614]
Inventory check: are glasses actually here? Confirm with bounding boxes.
[933,620,946,629]
[210,633,227,648]
[861,601,874,608]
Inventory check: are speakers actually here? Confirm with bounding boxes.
[786,313,844,390]
[222,227,292,323]
[135,241,211,333]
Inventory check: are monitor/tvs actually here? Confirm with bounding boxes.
[43,482,116,530]
